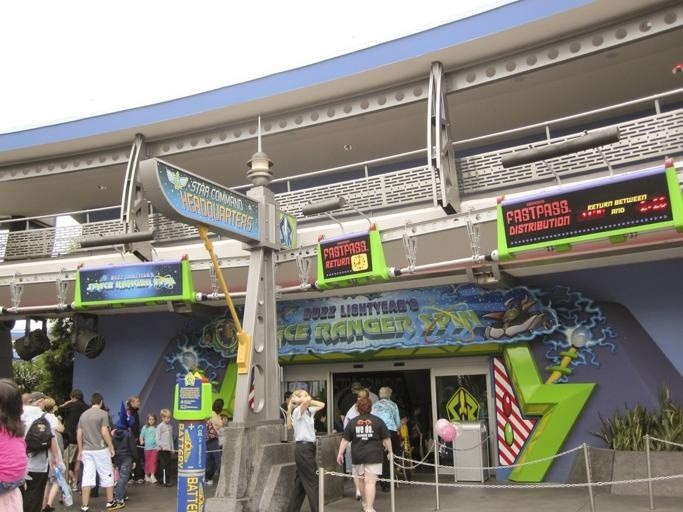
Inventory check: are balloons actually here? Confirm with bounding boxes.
[436,418,449,437]
[442,426,455,442]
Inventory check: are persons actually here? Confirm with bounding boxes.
[0,378,175,512]
[204,398,230,485]
[278,383,434,512]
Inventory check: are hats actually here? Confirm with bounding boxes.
[29,392,49,402]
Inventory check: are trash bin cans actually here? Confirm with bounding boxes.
[453,420,490,483]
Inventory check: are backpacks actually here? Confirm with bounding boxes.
[24,411,52,454]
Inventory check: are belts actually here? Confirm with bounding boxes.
[296,440,313,445]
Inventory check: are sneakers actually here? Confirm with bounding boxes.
[80,504,89,512]
[128,476,173,488]
[42,504,56,512]
[356,490,361,501]
[105,499,126,512]
